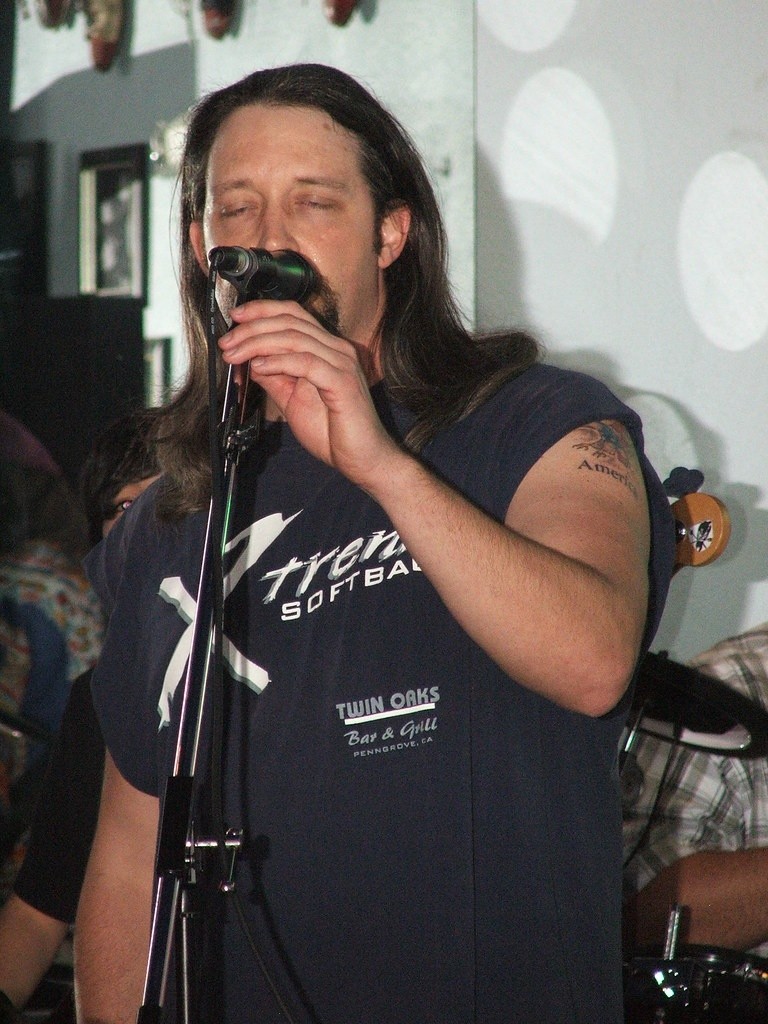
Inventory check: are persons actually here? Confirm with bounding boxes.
[622,622,768,981]
[71,63,678,1024]
[0,393,188,1024]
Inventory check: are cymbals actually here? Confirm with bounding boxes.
[623,650,768,759]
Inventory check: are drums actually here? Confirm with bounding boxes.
[623,936,768,1024]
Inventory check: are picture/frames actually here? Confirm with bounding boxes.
[74,143,149,309]
[141,338,171,410]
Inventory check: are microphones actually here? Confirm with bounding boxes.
[208,245,316,304]
